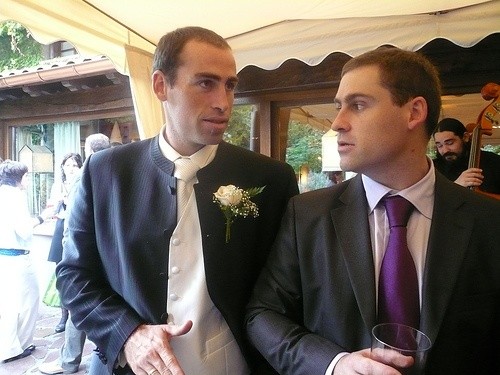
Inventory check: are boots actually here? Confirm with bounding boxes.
[55,308,69,333]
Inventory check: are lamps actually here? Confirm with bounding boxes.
[322,130,344,172]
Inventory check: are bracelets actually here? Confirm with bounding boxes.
[37,215,45,224]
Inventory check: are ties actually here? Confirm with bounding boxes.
[377,196,420,375]
[173,159,199,225]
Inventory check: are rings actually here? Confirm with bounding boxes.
[149,368,158,375]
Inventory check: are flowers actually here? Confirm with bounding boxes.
[212,184,267,243]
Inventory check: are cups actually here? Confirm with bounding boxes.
[371,322,432,375]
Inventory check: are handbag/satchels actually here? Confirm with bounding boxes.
[42,270,61,307]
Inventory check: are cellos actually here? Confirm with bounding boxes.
[458,83,500,198]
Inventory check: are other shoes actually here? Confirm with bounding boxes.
[38,359,64,374]
[4,345,35,363]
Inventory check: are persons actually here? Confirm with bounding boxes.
[431,117,500,198]
[55,26,304,375]
[244,46,500,375]
[0,134,123,375]
[325,171,346,188]
